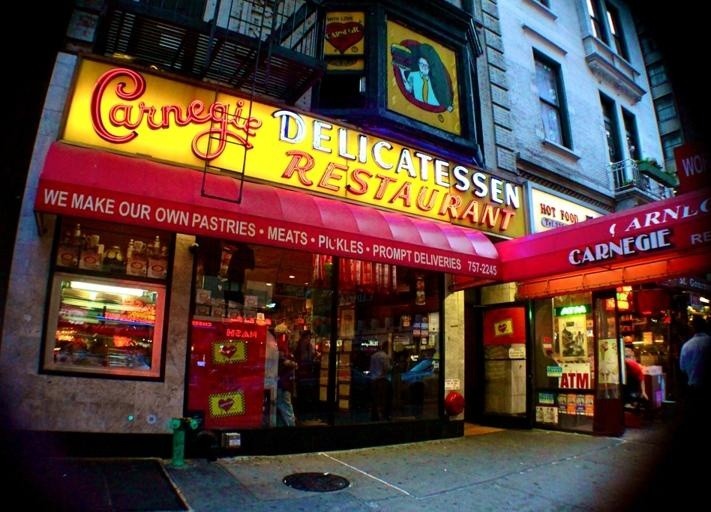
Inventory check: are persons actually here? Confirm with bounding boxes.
[679,318,711,395]
[366,341,399,422]
[401,348,436,383]
[624,358,649,409]
[294,330,317,380]
[399,58,441,106]
